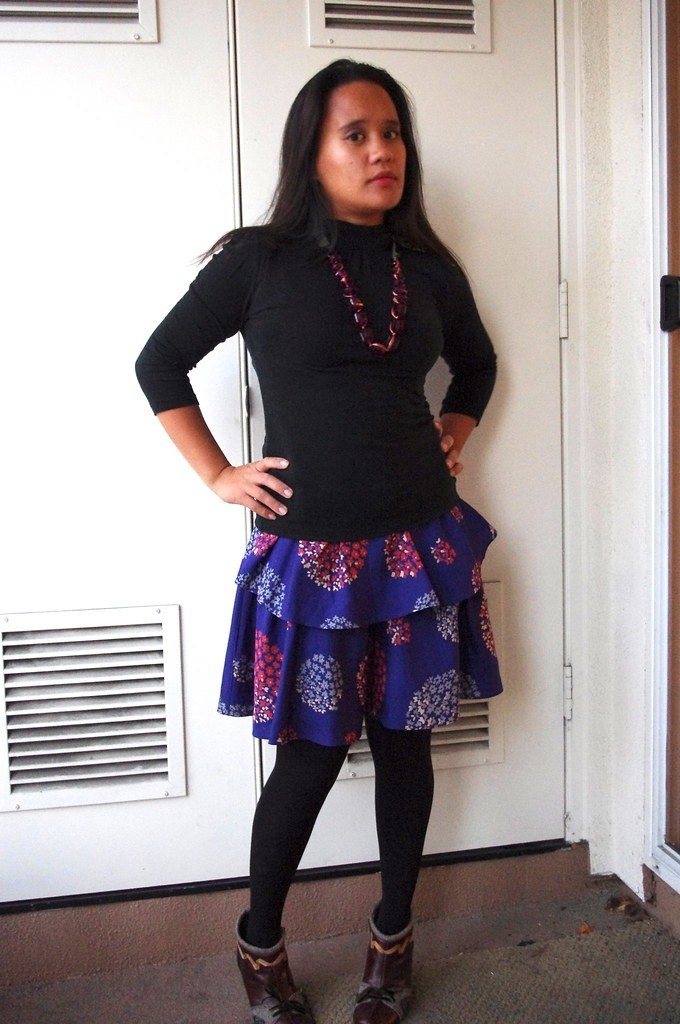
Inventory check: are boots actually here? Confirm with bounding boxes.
[235,909,314,1024]
[353,900,416,1024]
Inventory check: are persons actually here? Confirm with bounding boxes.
[134,60,504,1023]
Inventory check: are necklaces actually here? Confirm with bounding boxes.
[322,234,408,355]
